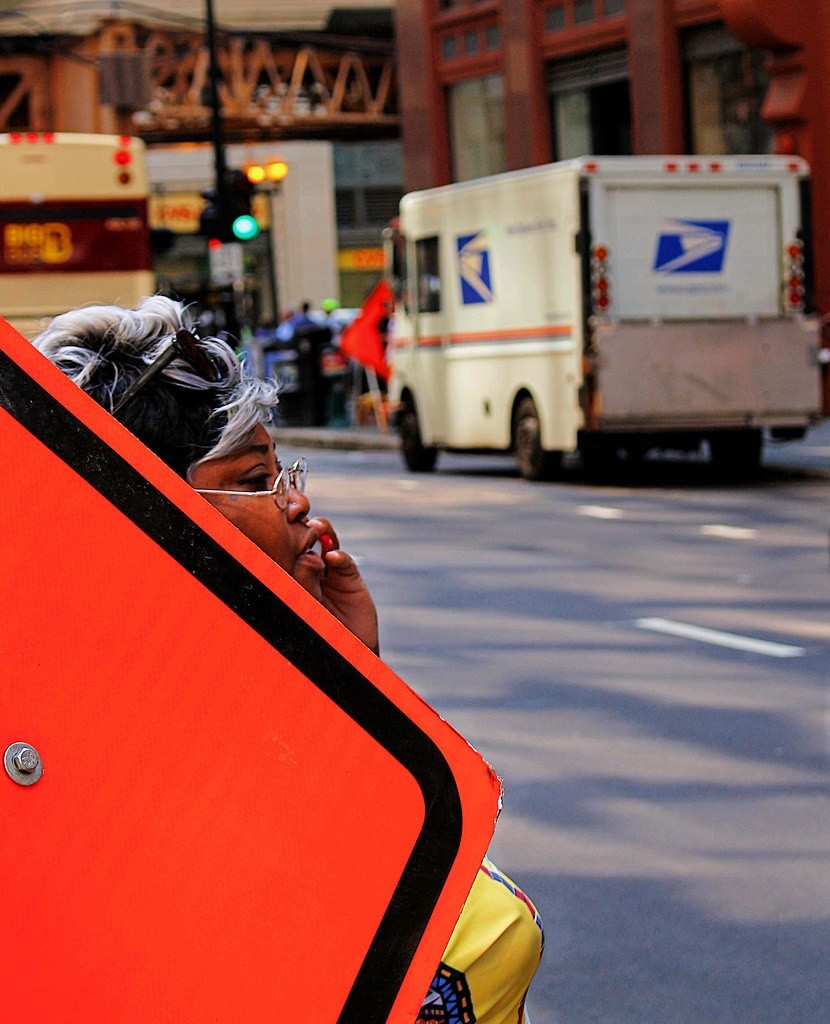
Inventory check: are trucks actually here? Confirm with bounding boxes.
[0,132,151,357]
[381,150,827,483]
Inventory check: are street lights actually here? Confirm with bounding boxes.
[247,159,289,324]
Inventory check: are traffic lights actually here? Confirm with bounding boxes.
[220,169,258,243]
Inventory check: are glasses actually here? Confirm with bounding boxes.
[193,456,309,511]
[106,319,224,419]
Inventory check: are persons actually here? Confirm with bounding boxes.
[11,295,549,1024]
[217,299,377,425]
